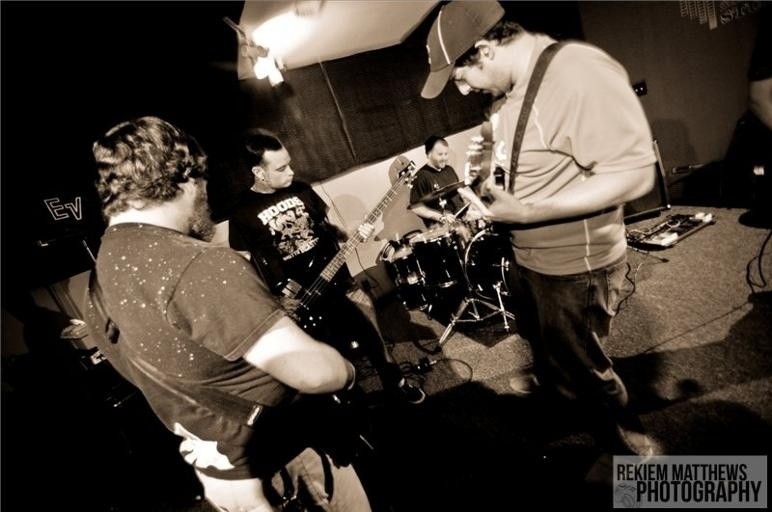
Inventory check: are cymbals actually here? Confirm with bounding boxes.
[407,181,465,209]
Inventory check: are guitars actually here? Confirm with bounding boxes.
[276,162,416,331]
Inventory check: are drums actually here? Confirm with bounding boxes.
[410,226,463,288]
[465,228,512,300]
[392,248,424,296]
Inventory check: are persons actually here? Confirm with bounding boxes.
[745,4,772,127]
[420,2,657,487]
[227,124,426,411]
[88,113,375,511]
[407,135,468,229]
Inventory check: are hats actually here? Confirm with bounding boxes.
[420,1,505,100]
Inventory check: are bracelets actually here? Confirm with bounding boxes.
[347,361,357,392]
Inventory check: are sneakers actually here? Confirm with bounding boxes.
[397,379,426,405]
[509,373,543,394]
